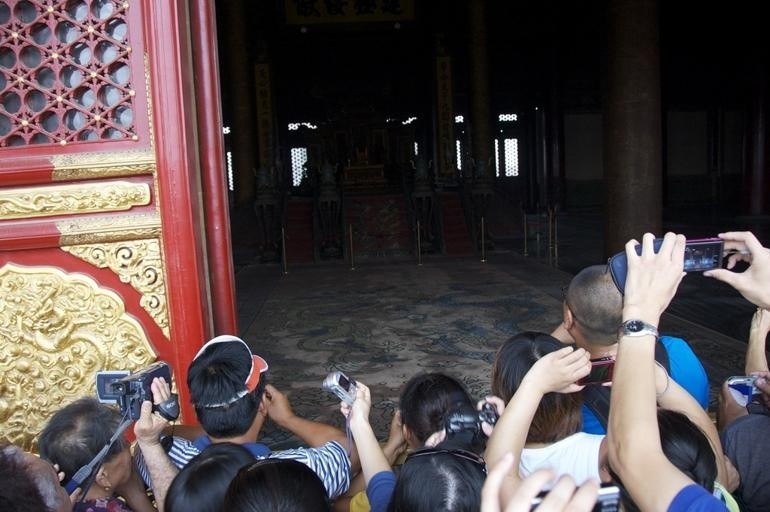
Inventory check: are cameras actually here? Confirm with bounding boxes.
[531,482,621,512]
[97,361,179,421]
[576,356,615,386]
[479,403,499,424]
[728,376,763,396]
[322,371,357,406]
[683,238,725,273]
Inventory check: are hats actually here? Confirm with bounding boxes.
[188,334,269,407]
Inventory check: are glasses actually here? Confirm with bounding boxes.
[561,286,576,321]
[405,448,485,468]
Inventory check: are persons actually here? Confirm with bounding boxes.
[3,306,769,512]
[606,230,768,510]
[550,265,709,412]
